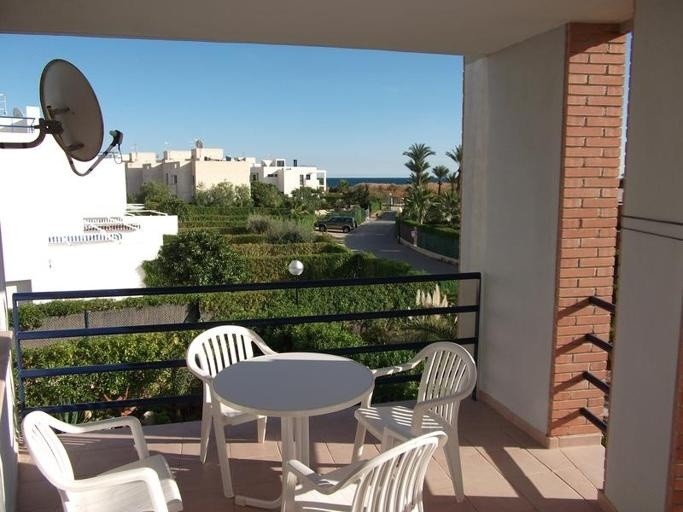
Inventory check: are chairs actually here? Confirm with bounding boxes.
[185,326,279,464]
[20,410,185,511]
[280,431,448,511]
[350,341,477,504]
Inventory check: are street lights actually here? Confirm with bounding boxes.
[288,260,304,305]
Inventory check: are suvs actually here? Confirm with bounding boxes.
[314,216,357,233]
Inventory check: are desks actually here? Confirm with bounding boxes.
[212,351,376,510]
